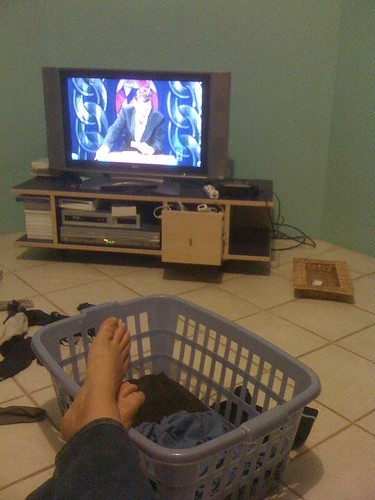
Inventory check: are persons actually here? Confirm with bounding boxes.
[23,316,165,500]
[96,87,165,159]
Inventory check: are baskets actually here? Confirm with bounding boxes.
[32,292,322,500]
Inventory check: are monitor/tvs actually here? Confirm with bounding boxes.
[42,67,231,179]
